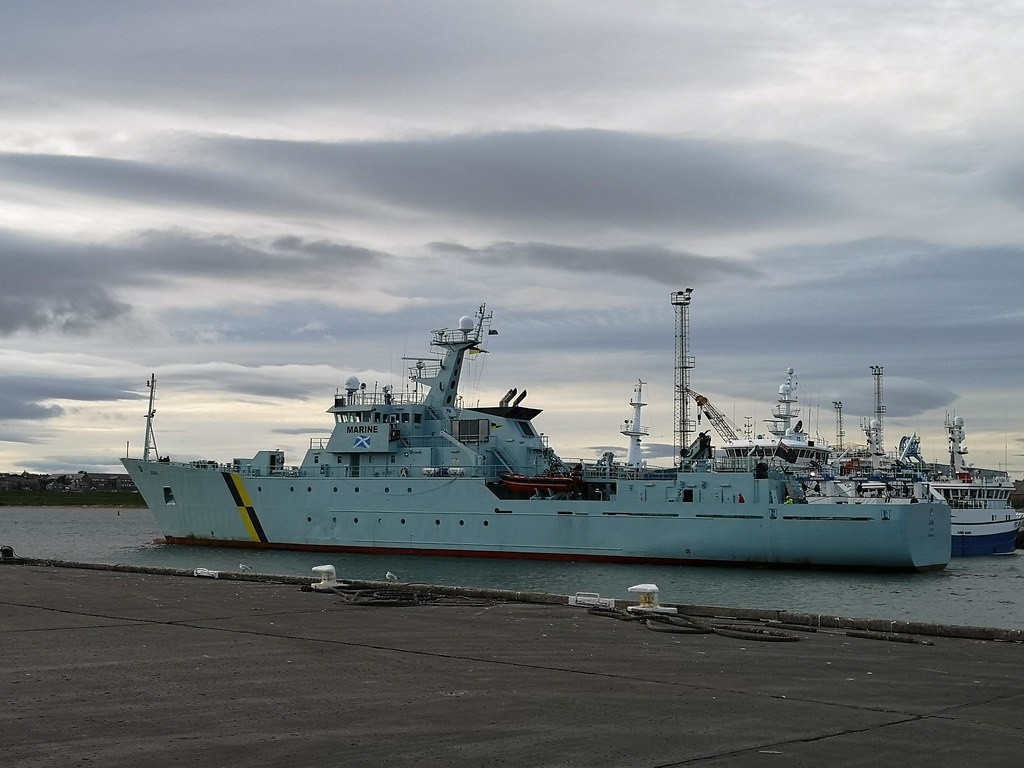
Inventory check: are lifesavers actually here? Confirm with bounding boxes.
[400,467,408,477]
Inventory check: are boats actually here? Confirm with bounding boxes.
[497,472,573,493]
[119,301,1023,576]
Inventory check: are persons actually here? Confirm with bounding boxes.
[909,495,920,505]
[783,494,794,504]
[736,494,746,504]
[157,455,173,464]
